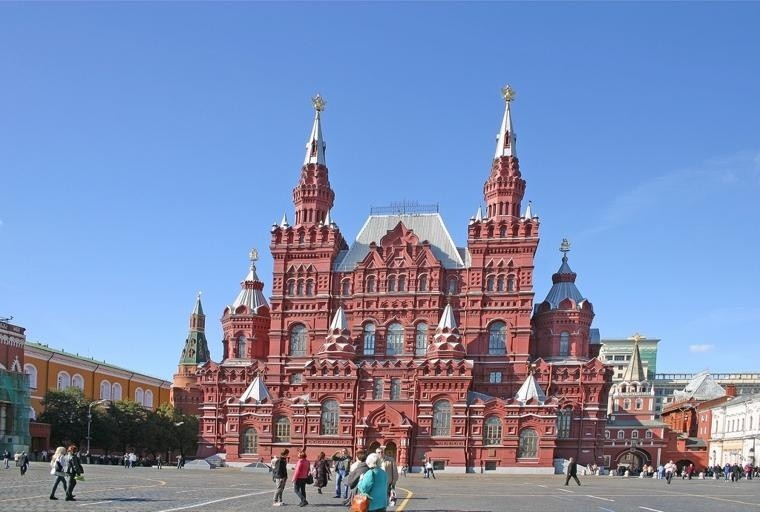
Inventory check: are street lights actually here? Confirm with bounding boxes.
[86,398,111,464]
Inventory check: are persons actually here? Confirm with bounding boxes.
[124,452,137,468]
[156,455,162,469]
[564,457,581,486]
[3,445,89,501]
[176,452,183,469]
[587,460,760,485]
[271,447,399,512]
[425,459,436,479]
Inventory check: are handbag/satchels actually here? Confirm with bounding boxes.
[307,472,313,484]
[351,495,369,512]
[70,465,83,473]
[310,467,318,476]
[55,461,63,472]
[389,489,396,507]
